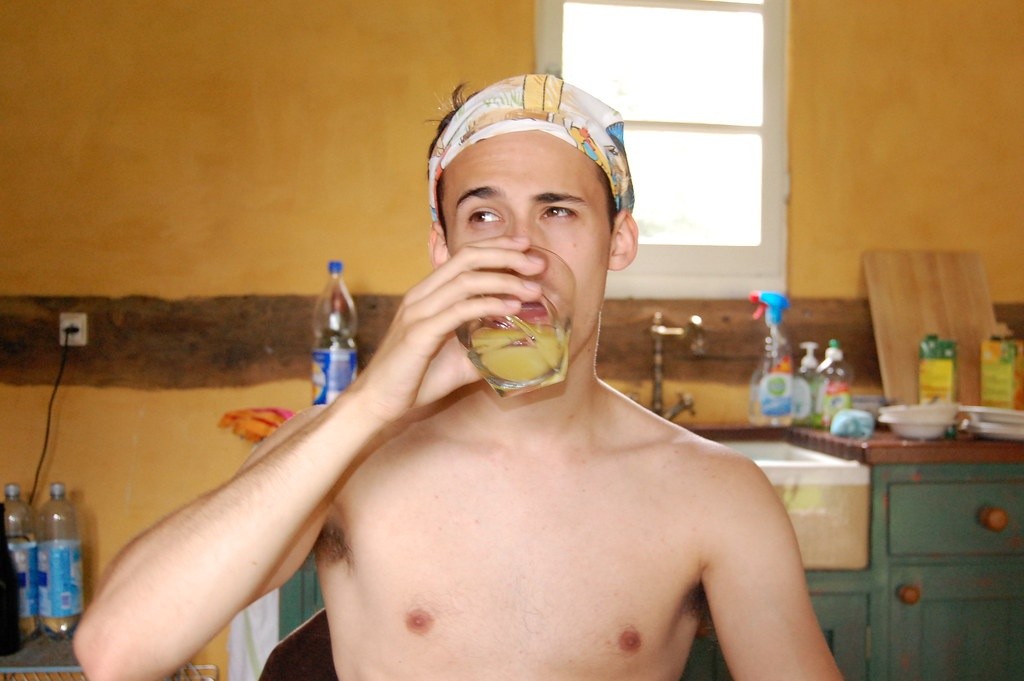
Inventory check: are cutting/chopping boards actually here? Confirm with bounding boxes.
[862,247,996,406]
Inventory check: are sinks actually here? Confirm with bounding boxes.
[715,440,871,570]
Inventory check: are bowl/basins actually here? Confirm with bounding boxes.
[876,405,956,439]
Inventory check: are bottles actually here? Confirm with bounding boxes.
[311,261,357,404]
[814,338,851,428]
[4,483,40,641]
[38,482,83,641]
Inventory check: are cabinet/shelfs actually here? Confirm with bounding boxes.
[279,459,1024,681]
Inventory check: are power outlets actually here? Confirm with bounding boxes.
[58,311,89,348]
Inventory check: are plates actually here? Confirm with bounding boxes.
[957,406,1024,441]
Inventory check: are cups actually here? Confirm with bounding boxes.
[454,243,575,398]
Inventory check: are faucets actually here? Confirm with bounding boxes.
[645,311,705,423]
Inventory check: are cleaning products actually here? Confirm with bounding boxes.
[745,290,852,431]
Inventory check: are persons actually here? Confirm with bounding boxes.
[69,74,849,681]
